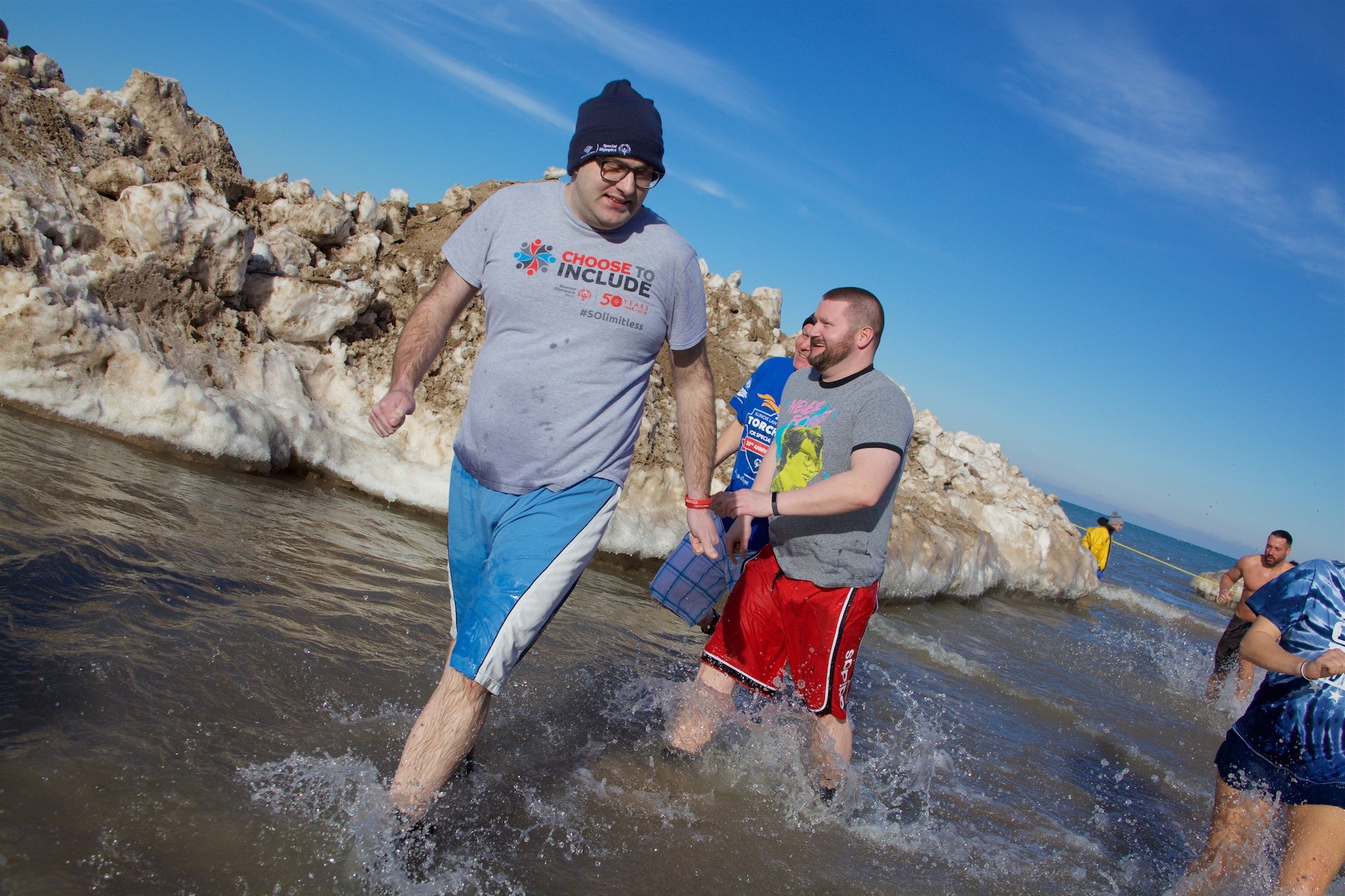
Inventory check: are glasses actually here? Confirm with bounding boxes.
[594,156,663,189]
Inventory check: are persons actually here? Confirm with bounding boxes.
[1078,510,1121,578]
[1202,529,1297,702]
[1166,556,1345,896]
[700,311,815,641]
[366,78,725,832]
[658,285,911,797]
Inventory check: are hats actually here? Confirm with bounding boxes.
[802,312,815,330]
[566,79,665,183]
[1107,511,1124,524]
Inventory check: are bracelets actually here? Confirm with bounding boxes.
[1302,662,1317,682]
[683,494,713,512]
[771,492,781,521]
